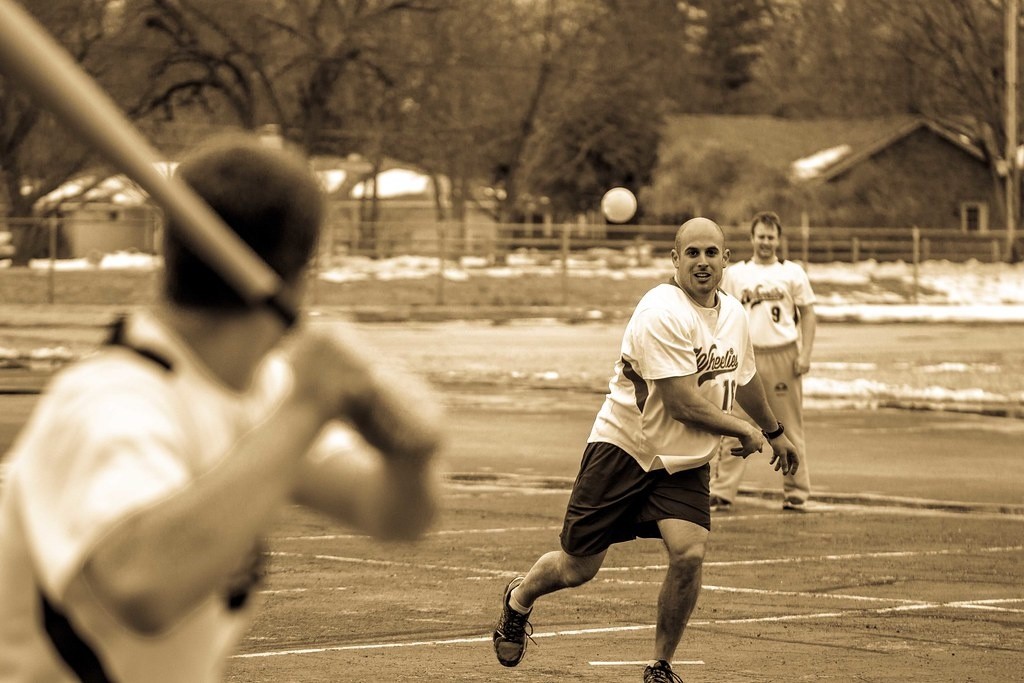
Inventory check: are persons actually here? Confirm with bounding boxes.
[710,210,819,508]
[492,218,801,683]
[0,135,441,683]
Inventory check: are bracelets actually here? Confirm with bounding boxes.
[761,422,785,440]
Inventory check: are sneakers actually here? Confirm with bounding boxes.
[493,575,539,667]
[643,659,683,683]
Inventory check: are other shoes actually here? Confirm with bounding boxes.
[783,496,808,509]
[709,495,729,511]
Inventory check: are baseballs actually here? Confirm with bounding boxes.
[601,187,637,224]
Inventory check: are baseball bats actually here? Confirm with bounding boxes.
[0,10,398,459]
